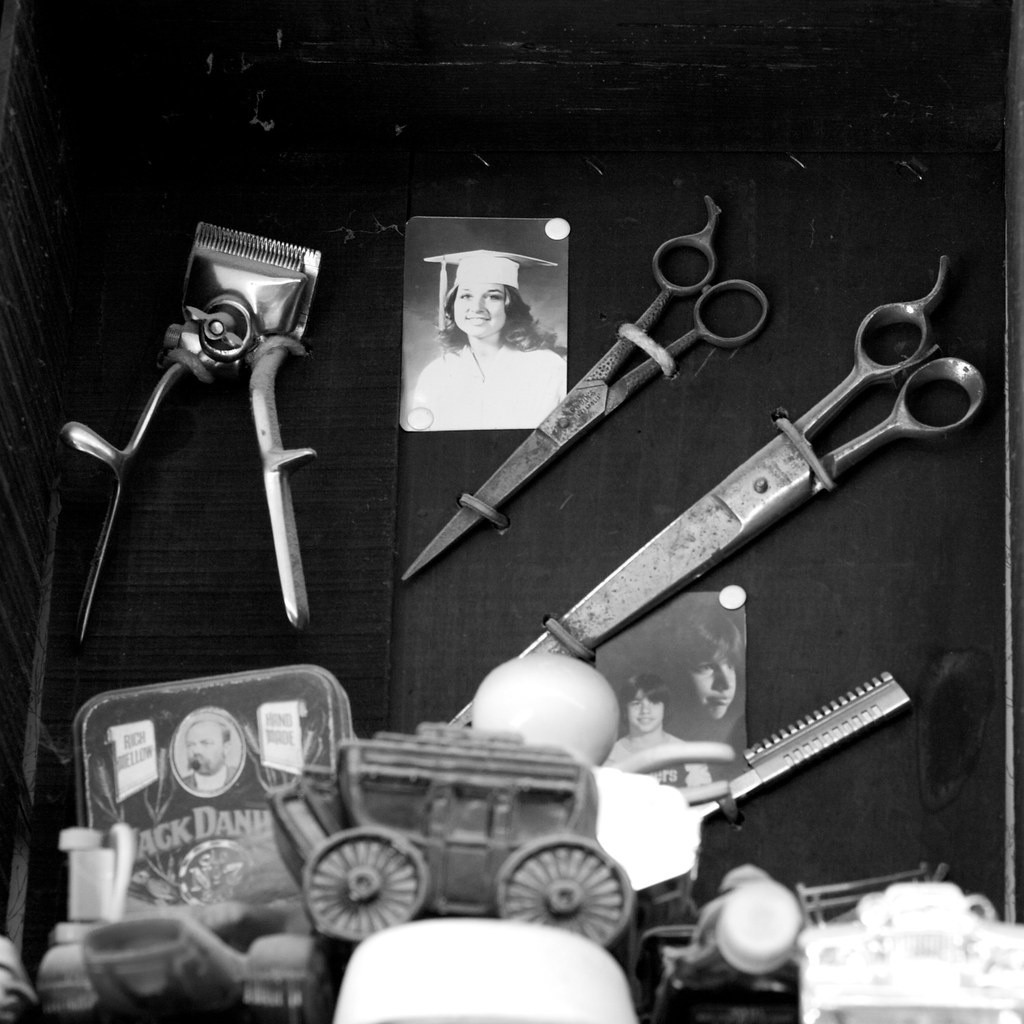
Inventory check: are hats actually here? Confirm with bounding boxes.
[424,249,558,331]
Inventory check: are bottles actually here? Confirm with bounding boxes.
[652,880,802,1024]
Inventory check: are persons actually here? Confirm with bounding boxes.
[183,719,238,790]
[602,607,743,785]
[408,251,566,427]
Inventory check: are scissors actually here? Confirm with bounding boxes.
[450,256,988,731]
[398,191,770,586]
